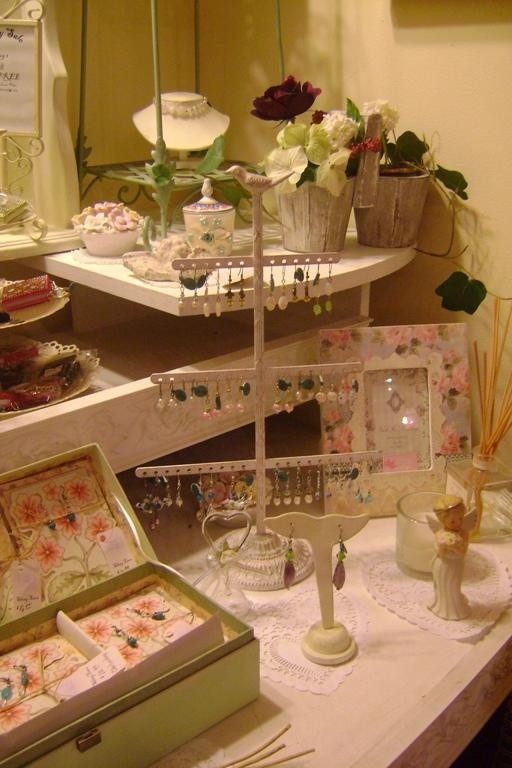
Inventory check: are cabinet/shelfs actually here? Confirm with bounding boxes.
[1,225,512,767]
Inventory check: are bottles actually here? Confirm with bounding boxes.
[203,552,255,619]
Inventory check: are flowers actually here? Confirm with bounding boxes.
[252,72,400,196]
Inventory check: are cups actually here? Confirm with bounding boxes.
[182,178,237,257]
[395,490,451,579]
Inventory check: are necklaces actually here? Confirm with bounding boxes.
[153,95,208,119]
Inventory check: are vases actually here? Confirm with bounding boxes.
[276,171,430,253]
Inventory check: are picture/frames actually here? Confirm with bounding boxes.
[319,323,473,518]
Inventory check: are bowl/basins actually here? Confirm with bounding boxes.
[74,223,142,257]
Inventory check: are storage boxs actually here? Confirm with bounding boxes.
[1,442,258,767]
